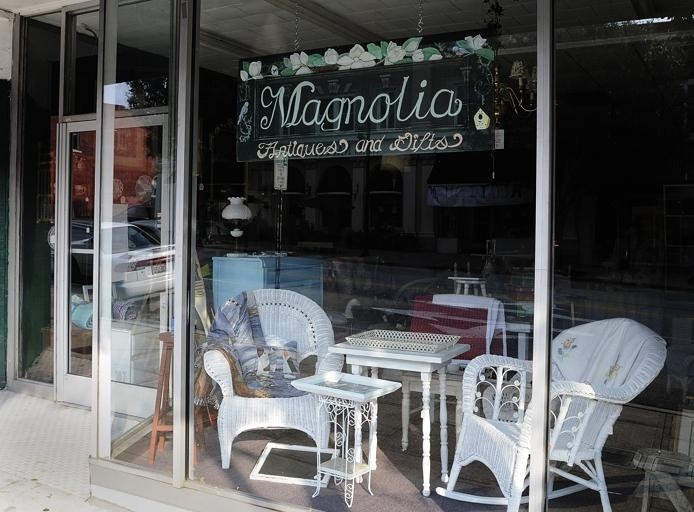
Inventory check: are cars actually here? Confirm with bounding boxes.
[68,206,236,305]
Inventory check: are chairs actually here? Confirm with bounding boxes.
[435,317,668,512]
[203,288,344,470]
[415,294,507,382]
[401,300,490,453]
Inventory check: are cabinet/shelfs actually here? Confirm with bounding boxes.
[111,322,160,388]
[290,370,402,508]
[212,255,324,315]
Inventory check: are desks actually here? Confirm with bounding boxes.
[506,320,534,360]
[327,341,472,497]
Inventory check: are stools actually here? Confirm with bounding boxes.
[148,331,216,465]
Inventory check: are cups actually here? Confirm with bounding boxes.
[325,371,341,383]
[74,184,87,196]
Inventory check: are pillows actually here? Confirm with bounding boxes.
[231,340,301,389]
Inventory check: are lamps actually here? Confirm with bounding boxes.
[494,1,537,118]
[220,197,253,258]
